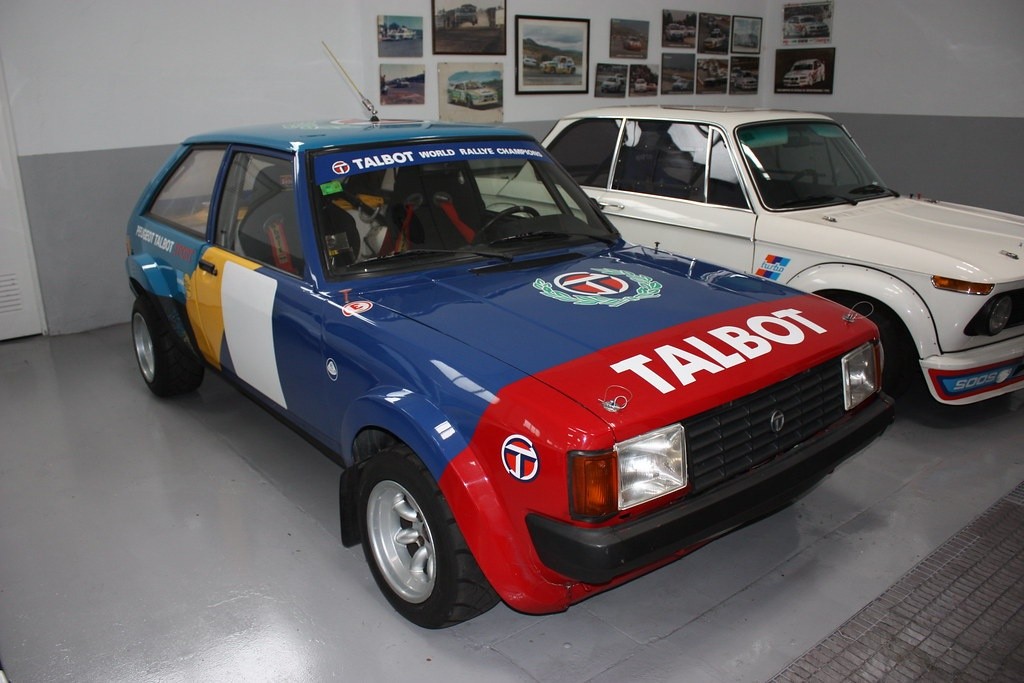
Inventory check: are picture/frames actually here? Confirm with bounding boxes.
[432,0,507,57]
[731,14,763,54]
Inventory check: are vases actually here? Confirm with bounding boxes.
[514,14,590,95]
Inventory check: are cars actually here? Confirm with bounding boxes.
[624,36,641,50]
[783,59,826,87]
[459,105,1024,406]
[524,55,537,67]
[601,76,621,93]
[665,23,684,42]
[704,31,726,49]
[397,78,410,88]
[452,81,498,108]
[734,70,757,89]
[125,120,900,629]
[784,15,828,37]
[540,55,576,75]
[634,79,647,92]
[672,78,690,91]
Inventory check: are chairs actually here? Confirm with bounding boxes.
[249,164,296,212]
[393,159,495,249]
[317,167,425,268]
[622,156,691,201]
